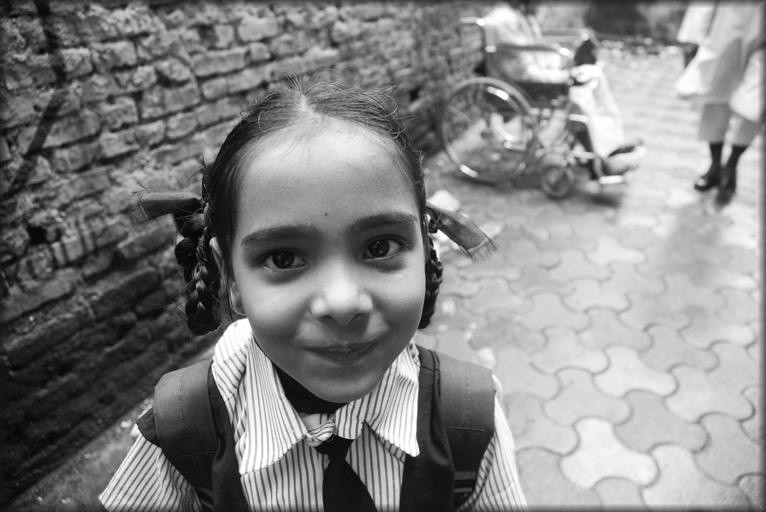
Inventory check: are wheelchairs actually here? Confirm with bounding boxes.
[436,16,642,196]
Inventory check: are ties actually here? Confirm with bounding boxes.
[316,435,376,512]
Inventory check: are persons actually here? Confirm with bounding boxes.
[98,79,527,511]
[671,1,766,203]
[482,1,646,176]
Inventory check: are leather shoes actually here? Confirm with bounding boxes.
[721,165,737,192]
[694,169,721,191]
